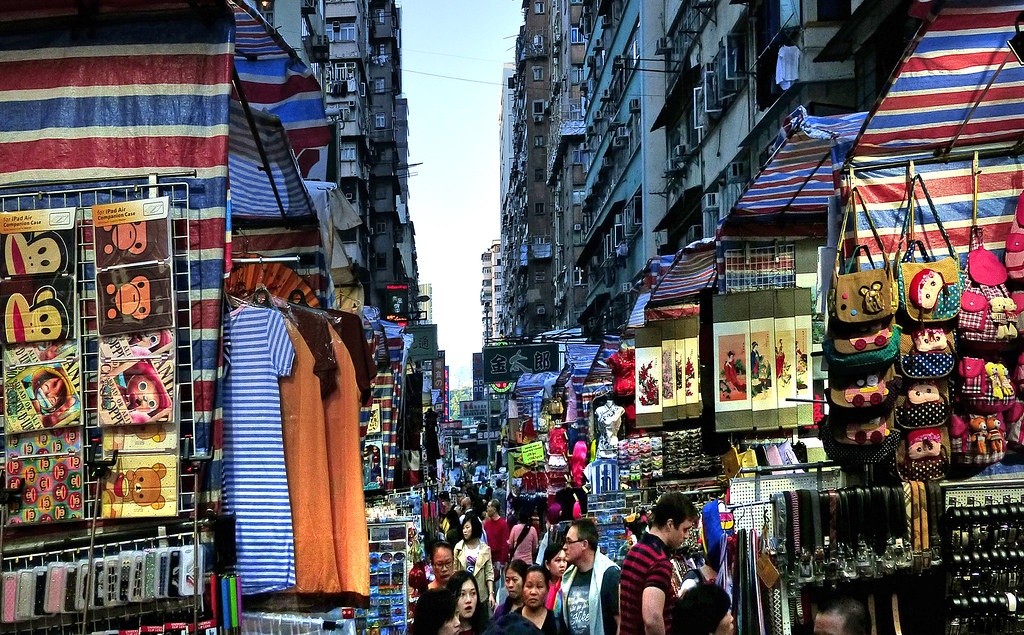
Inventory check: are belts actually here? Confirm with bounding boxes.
[947,502,1024,635]
[764,480,945,635]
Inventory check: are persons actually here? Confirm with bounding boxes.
[620,492,698,635]
[673,544,735,635]
[548,424,569,454]
[595,401,625,459]
[813,596,872,635]
[605,344,635,398]
[408,480,622,635]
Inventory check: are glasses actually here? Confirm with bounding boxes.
[562,536,580,544]
[432,559,454,569]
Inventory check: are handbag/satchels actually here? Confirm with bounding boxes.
[721,439,809,486]
[506,548,515,564]
[820,171,963,482]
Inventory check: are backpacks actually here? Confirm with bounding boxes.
[951,189,1024,467]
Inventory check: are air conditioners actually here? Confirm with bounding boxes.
[301,0,355,106]
[534,5,748,315]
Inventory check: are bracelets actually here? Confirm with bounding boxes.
[488,592,494,594]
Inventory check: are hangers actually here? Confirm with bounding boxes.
[224,267,313,312]
